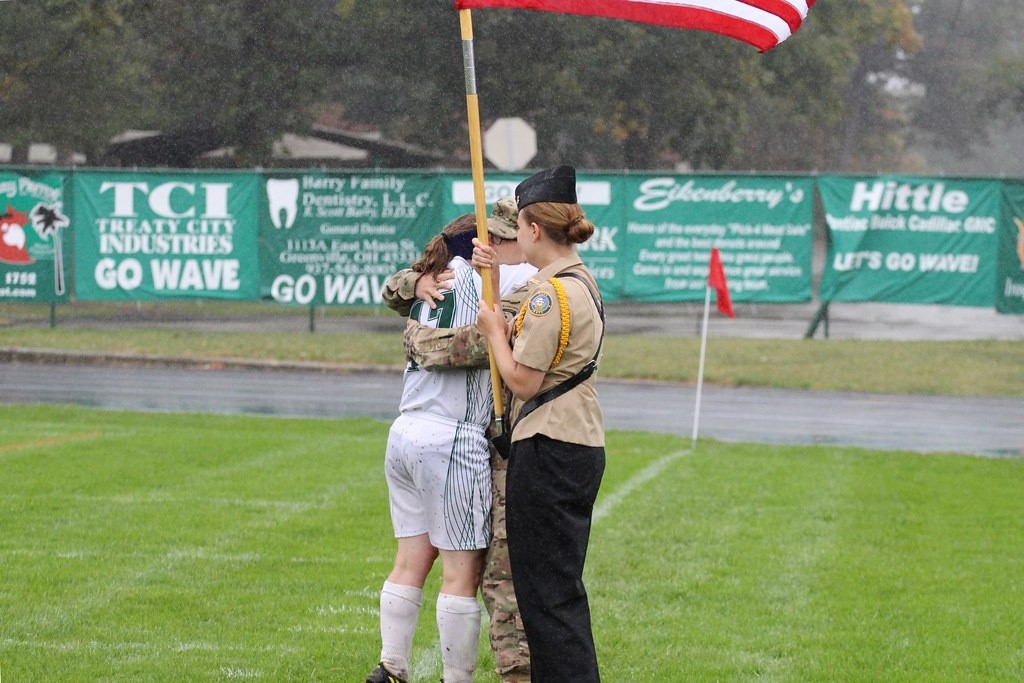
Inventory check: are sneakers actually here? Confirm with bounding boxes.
[366,661,410,683]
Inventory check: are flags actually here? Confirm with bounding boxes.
[708,248,735,317]
[454,1,815,54]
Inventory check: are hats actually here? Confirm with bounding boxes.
[474,197,520,239]
[515,165,577,211]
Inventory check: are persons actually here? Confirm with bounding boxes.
[365,212,504,683]
[381,194,531,683]
[477,165,606,683]
